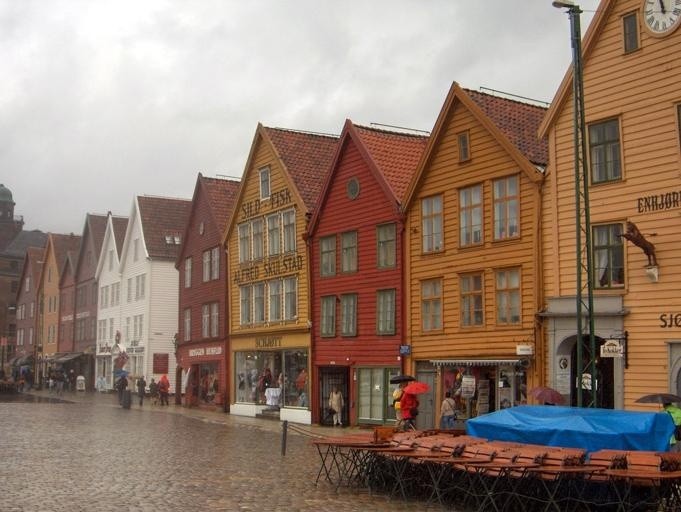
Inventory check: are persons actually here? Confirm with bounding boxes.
[393,381,403,424]
[327,387,346,426]
[399,388,419,432]
[439,391,457,427]
[0,358,171,406]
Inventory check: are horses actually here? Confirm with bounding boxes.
[616,221,658,266]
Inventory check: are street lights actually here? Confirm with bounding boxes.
[552,0,598,409]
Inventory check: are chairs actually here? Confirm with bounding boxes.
[313,428,681,511]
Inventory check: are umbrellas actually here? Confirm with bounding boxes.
[528,385,565,406]
[404,382,430,395]
[389,375,415,383]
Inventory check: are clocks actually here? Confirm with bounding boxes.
[641,0,681,37]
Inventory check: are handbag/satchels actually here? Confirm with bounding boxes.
[410,409,419,416]
[454,414,457,420]
